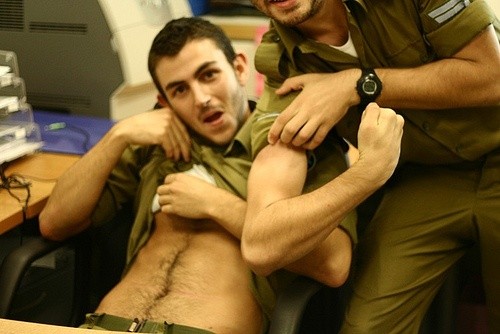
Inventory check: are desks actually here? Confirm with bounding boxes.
[0,150,85,236]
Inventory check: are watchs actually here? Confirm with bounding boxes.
[356,66,382,110]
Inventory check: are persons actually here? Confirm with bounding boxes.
[38,19,358,334]
[240,0,499,334]
